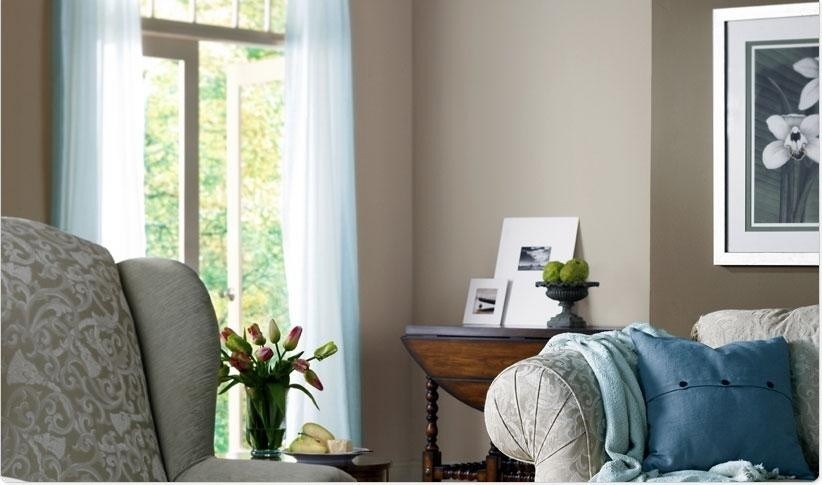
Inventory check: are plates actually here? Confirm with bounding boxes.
[282,447,372,461]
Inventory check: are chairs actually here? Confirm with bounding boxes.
[484,305,819,482]
[1,217,357,482]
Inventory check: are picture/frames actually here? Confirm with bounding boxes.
[710,2,820,268]
[462,277,510,328]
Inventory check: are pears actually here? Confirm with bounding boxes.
[542,261,563,283]
[561,259,589,284]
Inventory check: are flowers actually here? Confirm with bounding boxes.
[218,318,338,443]
[759,57,819,223]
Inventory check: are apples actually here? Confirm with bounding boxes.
[291,422,334,454]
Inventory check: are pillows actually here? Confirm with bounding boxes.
[1,217,169,482]
[690,305,819,466]
[629,327,815,480]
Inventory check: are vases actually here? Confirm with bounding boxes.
[244,382,288,458]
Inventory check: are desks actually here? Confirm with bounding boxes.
[224,457,393,482]
[400,324,623,482]
